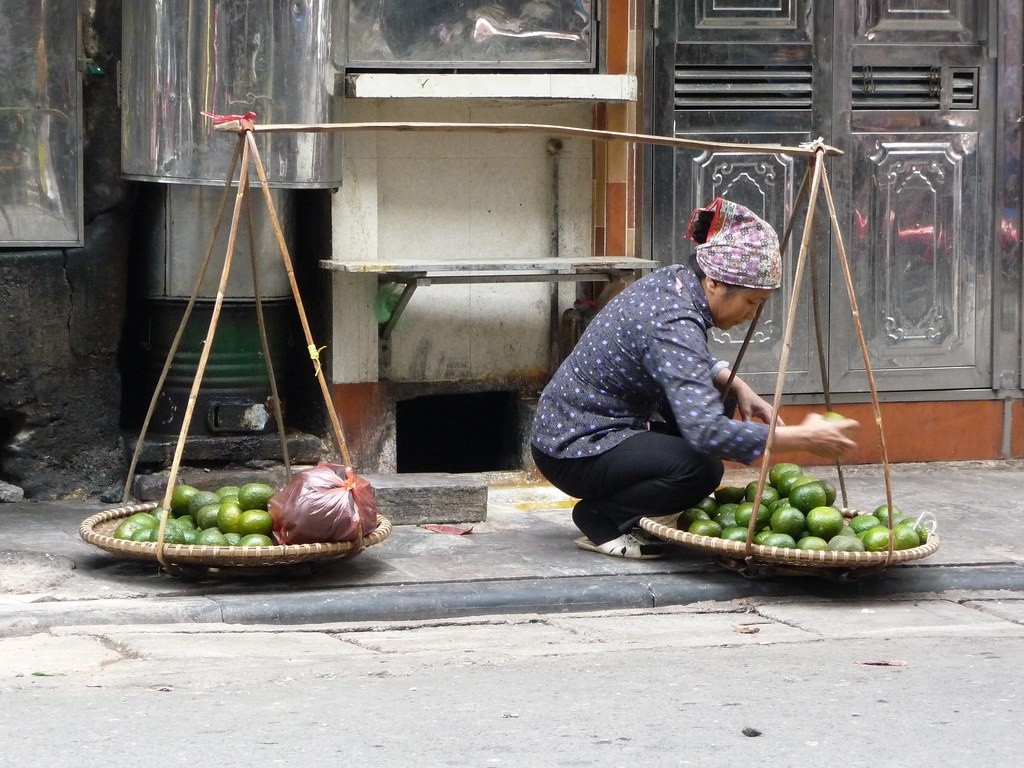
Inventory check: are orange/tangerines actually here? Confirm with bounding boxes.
[115,483,276,547]
[675,412,927,552]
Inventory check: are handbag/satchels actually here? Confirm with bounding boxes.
[269,456,380,546]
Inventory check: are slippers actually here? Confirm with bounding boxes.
[572,531,665,560]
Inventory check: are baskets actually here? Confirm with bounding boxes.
[77,497,393,568]
[638,504,939,579]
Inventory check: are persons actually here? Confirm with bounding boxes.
[530,197,861,560]
